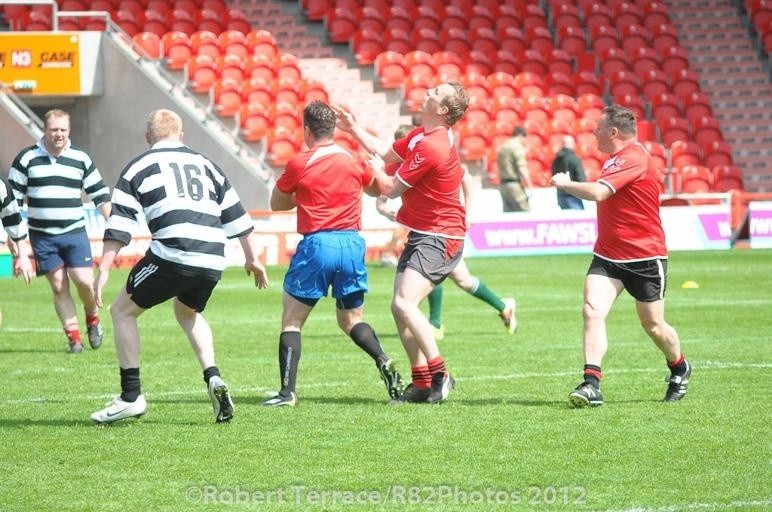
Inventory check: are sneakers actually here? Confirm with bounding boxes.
[663,358,691,402]
[569,381,604,407]
[85,316,103,349]
[90,393,148,423]
[262,392,298,407]
[386,383,428,405]
[207,376,236,423]
[67,340,84,353]
[428,322,444,342]
[376,354,405,399]
[499,295,518,335]
[427,373,457,404]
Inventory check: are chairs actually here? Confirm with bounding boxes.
[527,0,541,8]
[678,164,713,193]
[572,71,602,98]
[214,78,245,116]
[661,200,687,206]
[165,8,197,37]
[619,24,650,56]
[526,161,545,189]
[583,4,610,27]
[410,27,443,54]
[693,115,724,147]
[578,94,605,120]
[489,119,514,147]
[448,0,473,10]
[628,48,657,81]
[750,0,772,30]
[640,68,671,105]
[273,78,300,106]
[515,71,546,97]
[438,5,468,31]
[471,27,499,54]
[526,26,554,55]
[635,119,657,142]
[642,3,670,29]
[409,7,442,31]
[607,1,630,10]
[461,71,489,98]
[547,48,573,79]
[670,67,700,101]
[0,5,27,24]
[245,79,274,106]
[189,28,221,58]
[434,52,464,76]
[301,0,332,22]
[188,53,218,94]
[613,3,640,30]
[635,1,659,7]
[492,96,523,124]
[137,8,166,39]
[614,95,644,122]
[496,6,522,32]
[79,15,105,31]
[168,0,197,15]
[327,7,356,43]
[487,151,500,188]
[499,28,527,57]
[551,95,580,119]
[196,7,223,36]
[553,4,578,27]
[544,73,575,98]
[404,50,434,77]
[355,7,386,32]
[335,0,359,12]
[609,70,640,96]
[249,30,280,59]
[217,30,247,61]
[31,5,53,18]
[374,51,407,89]
[524,5,547,36]
[524,119,547,148]
[433,74,453,88]
[467,7,494,30]
[132,31,161,61]
[599,46,629,77]
[651,92,682,122]
[272,52,301,81]
[643,141,667,169]
[364,0,391,10]
[576,0,603,8]
[162,30,192,70]
[670,140,701,171]
[650,22,679,47]
[522,96,549,123]
[404,74,432,114]
[58,16,79,30]
[486,72,517,97]
[548,0,572,9]
[590,24,620,54]
[523,144,546,172]
[57,1,83,11]
[463,96,491,128]
[440,27,471,55]
[111,0,141,12]
[727,189,748,225]
[713,165,744,192]
[384,27,410,54]
[21,11,48,30]
[574,120,598,157]
[703,140,732,172]
[683,93,713,123]
[659,43,690,78]
[557,22,586,58]
[271,103,302,130]
[244,54,275,81]
[760,20,772,51]
[111,10,141,37]
[332,130,358,153]
[475,0,500,13]
[87,0,112,10]
[458,125,489,161]
[503,1,527,15]
[385,6,412,31]
[222,8,251,37]
[196,0,226,17]
[548,119,577,153]
[219,54,244,81]
[266,127,299,157]
[239,103,271,142]
[137,0,170,13]
[462,50,493,78]
[420,0,449,7]
[391,0,419,9]
[577,52,596,74]
[518,50,548,78]
[660,116,689,148]
[577,144,602,183]
[353,27,384,66]
[491,50,518,75]
[299,77,329,107]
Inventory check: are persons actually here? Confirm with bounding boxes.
[496,126,532,212]
[332,81,467,403]
[0,175,36,285]
[6,108,111,352]
[88,106,270,423]
[376,124,519,340]
[550,105,693,408]
[264,103,404,405]
[552,134,586,210]
